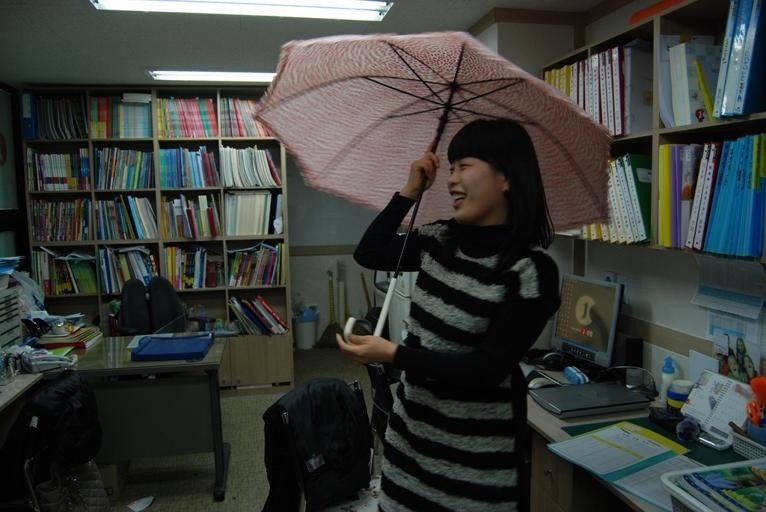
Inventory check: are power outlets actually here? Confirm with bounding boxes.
[604,272,629,305]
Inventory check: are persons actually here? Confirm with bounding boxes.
[335,118,562,512]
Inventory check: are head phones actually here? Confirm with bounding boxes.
[522,347,564,366]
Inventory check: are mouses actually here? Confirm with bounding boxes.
[527,377,558,390]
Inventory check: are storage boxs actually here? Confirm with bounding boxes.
[731,428,766,460]
[660,458,766,512]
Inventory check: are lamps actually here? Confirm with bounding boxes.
[92,0,394,21]
[147,70,274,81]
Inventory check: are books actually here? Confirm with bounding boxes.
[38,326,103,348]
[92,93,153,139]
[30,199,91,242]
[227,240,286,287]
[158,98,218,137]
[228,294,289,336]
[161,194,220,238]
[681,369,753,434]
[659,0,766,129]
[95,145,154,188]
[659,134,765,257]
[23,93,85,140]
[580,153,650,244]
[226,190,283,235]
[222,146,280,186]
[165,246,224,288]
[27,148,88,191]
[95,195,157,239]
[670,460,766,512]
[99,246,158,294]
[160,147,220,187]
[545,37,653,136]
[33,247,97,295]
[220,98,275,136]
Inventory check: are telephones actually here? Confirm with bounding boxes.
[21,351,79,375]
[27,354,78,373]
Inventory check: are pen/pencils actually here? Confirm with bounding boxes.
[728,421,746,436]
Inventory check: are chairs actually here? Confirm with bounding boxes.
[349,320,404,451]
[264,377,385,512]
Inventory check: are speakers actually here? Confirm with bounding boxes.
[608,332,642,386]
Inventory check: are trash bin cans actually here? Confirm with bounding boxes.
[294,308,317,349]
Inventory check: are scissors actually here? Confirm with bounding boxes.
[747,400,761,427]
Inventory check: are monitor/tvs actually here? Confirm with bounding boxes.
[551,273,625,375]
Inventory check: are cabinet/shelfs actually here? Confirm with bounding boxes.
[14,83,294,386]
[540,0,765,263]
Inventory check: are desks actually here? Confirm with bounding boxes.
[520,353,766,512]
[0,314,104,511]
[69,337,228,503]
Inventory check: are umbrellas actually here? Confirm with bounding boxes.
[252,31,614,346]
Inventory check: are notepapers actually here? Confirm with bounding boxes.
[713,333,729,356]
